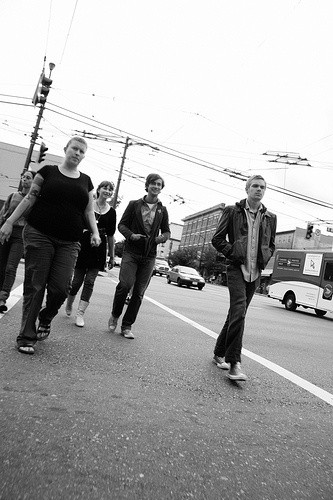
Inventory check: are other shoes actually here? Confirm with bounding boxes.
[0,301,8,312]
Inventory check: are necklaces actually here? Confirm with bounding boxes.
[97,203,100,209]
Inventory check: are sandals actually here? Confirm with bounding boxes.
[18,343,35,354]
[37,324,50,340]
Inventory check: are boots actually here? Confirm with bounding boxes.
[66,297,74,316]
[75,299,89,327]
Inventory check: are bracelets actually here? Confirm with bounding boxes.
[160,235,164,238]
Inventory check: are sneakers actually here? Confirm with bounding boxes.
[121,329,135,338]
[212,355,230,369]
[108,316,117,330]
[225,366,248,381]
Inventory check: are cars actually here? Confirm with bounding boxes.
[150,255,171,277]
[165,264,206,290]
[106,255,122,266]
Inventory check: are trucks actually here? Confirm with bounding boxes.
[266,248,333,317]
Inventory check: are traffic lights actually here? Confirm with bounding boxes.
[31,72,53,106]
[304,223,314,239]
[37,141,49,164]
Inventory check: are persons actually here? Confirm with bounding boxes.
[0,172,35,311]
[212,175,277,381]
[0,138,101,355]
[107,174,171,339]
[66,181,116,327]
